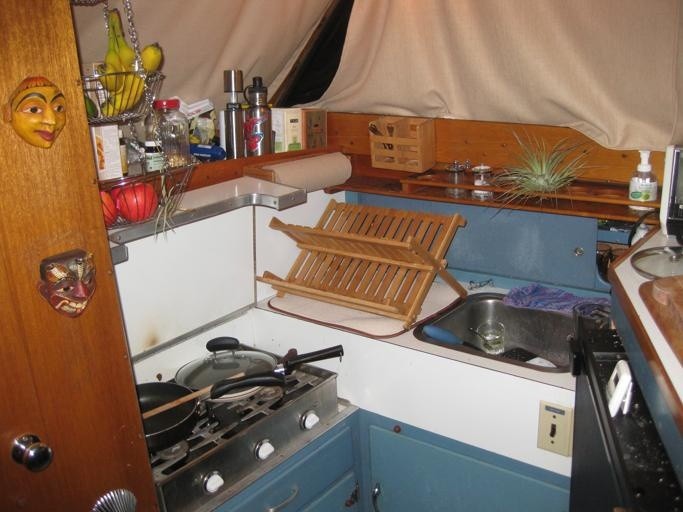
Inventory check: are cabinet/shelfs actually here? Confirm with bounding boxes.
[97,100,669,244]
[214,409,362,510]
[365,416,571,512]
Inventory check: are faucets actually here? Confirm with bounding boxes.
[573,301,613,332]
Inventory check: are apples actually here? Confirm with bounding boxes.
[111,183,134,216]
[99,190,116,227]
[119,182,159,224]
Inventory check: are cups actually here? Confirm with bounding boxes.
[477,320,505,355]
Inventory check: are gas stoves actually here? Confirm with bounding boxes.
[150,343,339,511]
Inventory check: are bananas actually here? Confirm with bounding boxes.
[101,73,146,116]
[99,9,163,91]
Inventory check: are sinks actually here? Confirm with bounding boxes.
[413,292,610,374]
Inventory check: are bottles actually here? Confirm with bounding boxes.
[118,129,128,176]
[153,99,189,169]
[245,76,271,160]
[144,139,163,171]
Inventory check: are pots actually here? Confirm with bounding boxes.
[174,336,345,403]
[136,372,285,454]
